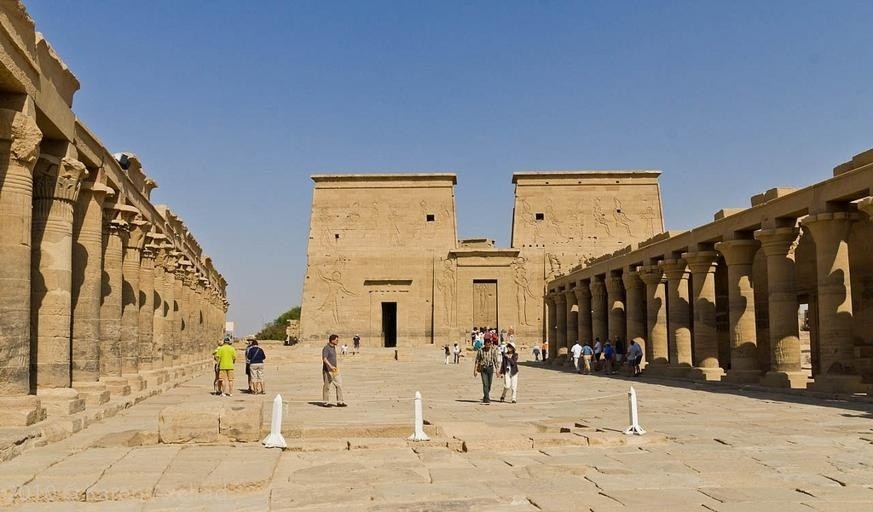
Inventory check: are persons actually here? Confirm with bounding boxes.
[353,335,360,353]
[473,339,499,404]
[454,341,460,364]
[322,334,347,407]
[341,345,348,355]
[285,335,297,346]
[472,326,498,348]
[497,343,518,403]
[532,346,540,362]
[442,344,449,364]
[560,337,643,377]
[213,338,266,397]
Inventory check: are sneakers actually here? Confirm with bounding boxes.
[248,389,266,395]
[322,402,332,407]
[210,390,233,397]
[483,396,491,403]
[512,399,516,403]
[336,402,348,408]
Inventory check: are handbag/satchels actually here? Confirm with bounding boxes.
[246,363,250,375]
[599,351,606,360]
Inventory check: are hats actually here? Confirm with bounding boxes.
[605,341,609,344]
[246,335,256,342]
[508,342,516,349]
